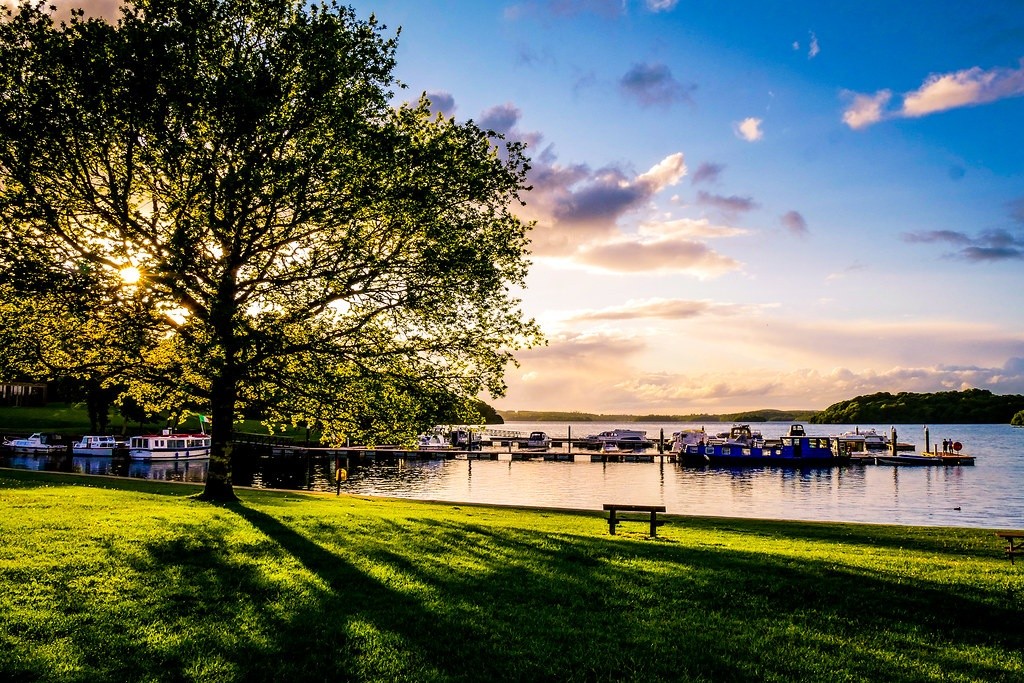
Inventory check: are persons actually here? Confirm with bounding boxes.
[88,438,92,449]
[942,438,954,457]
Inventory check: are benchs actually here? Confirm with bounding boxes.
[603,504,666,537]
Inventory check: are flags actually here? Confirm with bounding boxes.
[199,415,211,423]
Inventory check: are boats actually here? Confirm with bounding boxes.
[527,432,553,446]
[72,434,132,457]
[577,428,652,449]
[412,427,483,451]
[128,427,212,460]
[676,434,854,468]
[1,431,68,454]
[662,422,887,452]
